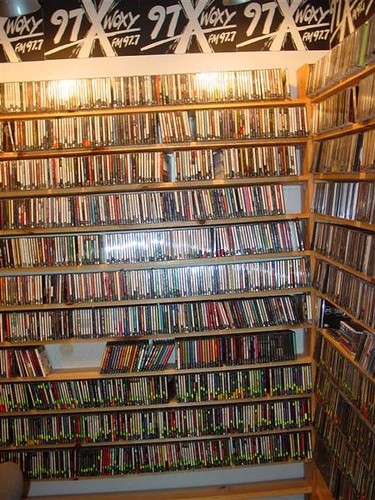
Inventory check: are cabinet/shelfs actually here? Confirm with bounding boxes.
[304,15,375,500]
[0,61,315,500]
